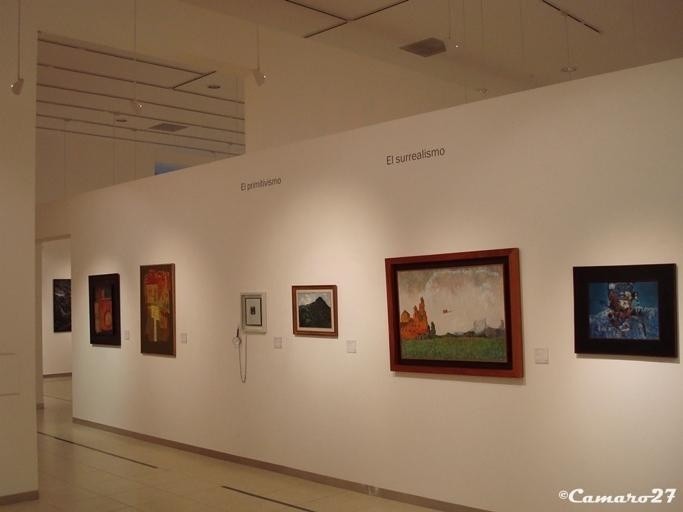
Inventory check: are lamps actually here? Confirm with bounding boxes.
[251,10,266,84]
[130,0,144,114]
[10,0,25,97]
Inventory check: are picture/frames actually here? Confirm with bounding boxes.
[240,291,267,334]
[384,248,523,378]
[570,261,682,361]
[292,285,338,337]
